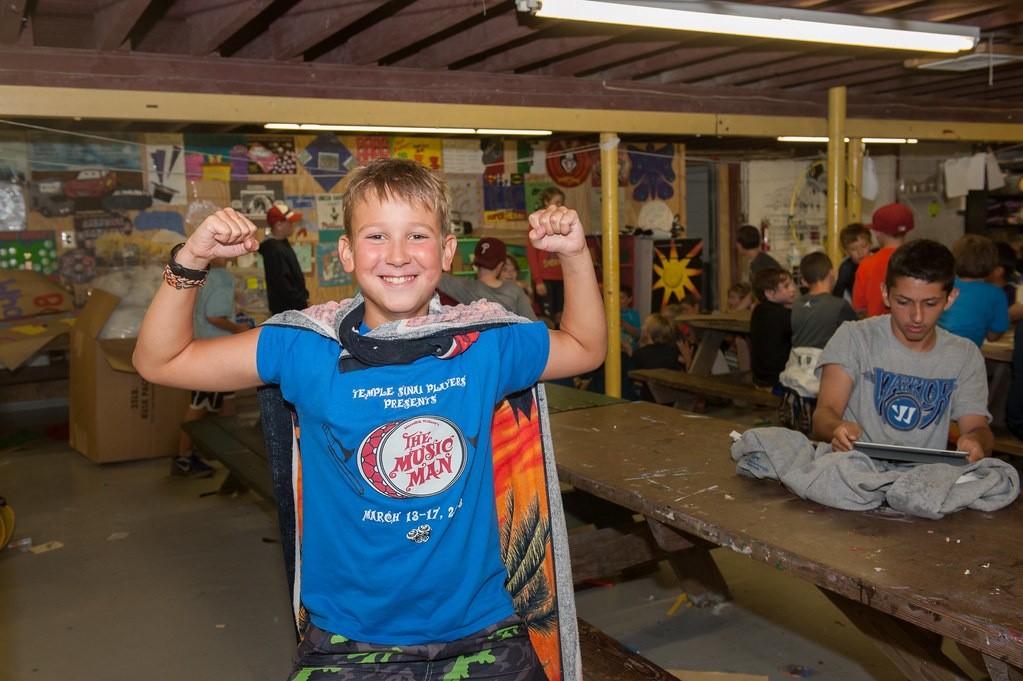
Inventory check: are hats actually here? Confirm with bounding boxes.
[265,201,303,227]
[473,238,507,269]
[869,202,915,237]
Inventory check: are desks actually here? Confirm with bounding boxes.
[543,384,628,416]
[673,306,1015,420]
[548,401,1023,681]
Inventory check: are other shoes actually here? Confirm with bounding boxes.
[778,392,795,429]
[796,401,811,434]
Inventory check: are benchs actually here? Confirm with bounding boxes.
[575,616,684,681]
[622,368,1023,460]
[179,415,274,507]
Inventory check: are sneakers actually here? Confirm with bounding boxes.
[169,453,216,480]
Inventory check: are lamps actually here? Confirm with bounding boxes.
[514,0,979,55]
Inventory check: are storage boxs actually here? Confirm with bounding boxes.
[0,257,239,466]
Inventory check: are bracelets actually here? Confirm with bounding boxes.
[162,241,208,289]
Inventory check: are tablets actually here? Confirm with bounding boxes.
[853,441,970,457]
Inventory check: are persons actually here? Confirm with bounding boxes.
[437,187,1023,472]
[174,258,253,479]
[130,156,608,681]
[258,200,310,315]
